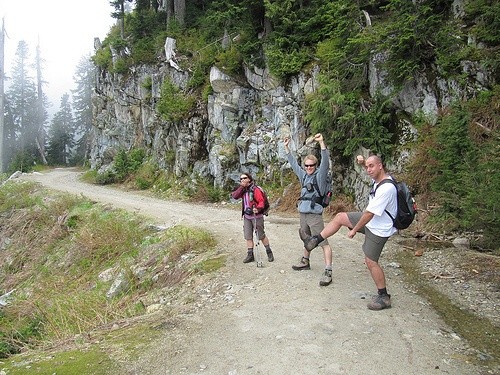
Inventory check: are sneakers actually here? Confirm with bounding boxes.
[292,257,310,270]
[244,252,254,262]
[319,269,332,285]
[299,228,318,252]
[266,248,274,261]
[367,294,391,311]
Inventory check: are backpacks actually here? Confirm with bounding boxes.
[302,172,332,208]
[250,185,269,215]
[372,175,415,230]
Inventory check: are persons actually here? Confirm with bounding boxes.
[299,155,398,310]
[232,173,274,263]
[284,133,332,286]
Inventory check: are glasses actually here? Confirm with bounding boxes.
[305,163,316,167]
[240,177,248,180]
